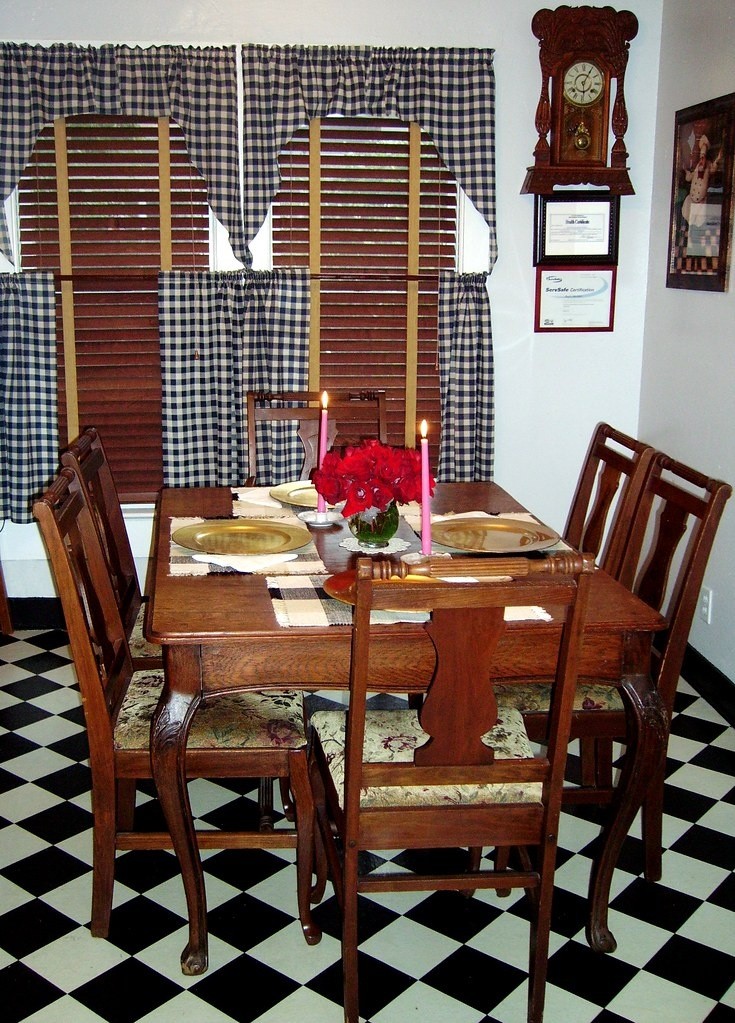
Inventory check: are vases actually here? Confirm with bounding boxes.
[345,498,400,547]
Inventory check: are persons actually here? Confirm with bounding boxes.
[682,136,723,226]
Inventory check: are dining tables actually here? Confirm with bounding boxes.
[142,481,667,978]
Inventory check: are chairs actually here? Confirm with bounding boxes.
[33,389,727,1022]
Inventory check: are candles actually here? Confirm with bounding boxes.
[419,418,432,556]
[317,391,328,513]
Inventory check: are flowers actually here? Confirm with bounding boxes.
[305,434,437,524]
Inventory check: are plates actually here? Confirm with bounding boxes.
[430,518,560,553]
[173,520,313,554]
[323,569,449,610]
[269,480,337,508]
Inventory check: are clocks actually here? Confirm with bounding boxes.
[561,58,605,107]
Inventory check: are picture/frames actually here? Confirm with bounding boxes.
[665,91,735,293]
[536,265,615,331]
[537,190,621,265]
